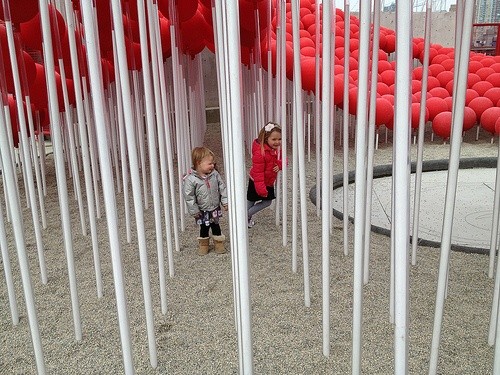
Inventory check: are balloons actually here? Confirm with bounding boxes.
[0,0,500,147]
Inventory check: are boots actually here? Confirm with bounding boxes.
[197,235,226,256]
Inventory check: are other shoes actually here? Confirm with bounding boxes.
[248,219,255,228]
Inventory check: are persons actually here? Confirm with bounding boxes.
[247,122,288,228]
[181,147,228,256]
